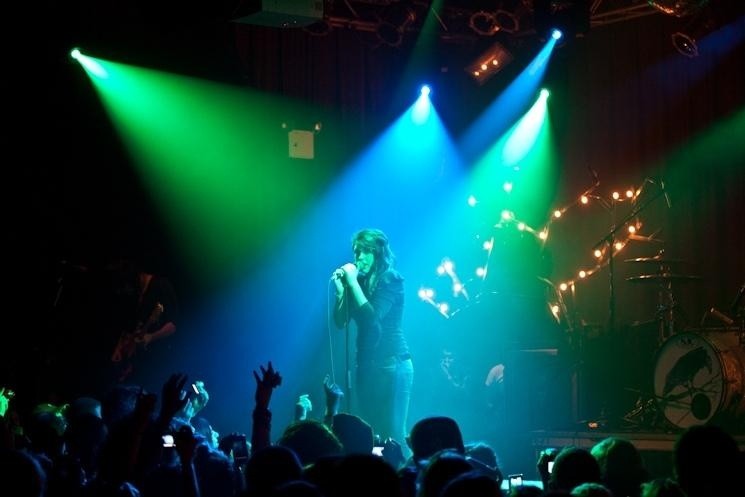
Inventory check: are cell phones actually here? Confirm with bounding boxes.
[372,446,385,457]
[546,461,555,473]
[508,474,524,494]
[162,432,176,447]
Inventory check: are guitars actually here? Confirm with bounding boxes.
[113,303,164,380]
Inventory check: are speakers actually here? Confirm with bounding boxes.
[503,348,557,434]
[228,0,324,28]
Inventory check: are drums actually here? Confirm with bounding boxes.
[651,330,745,427]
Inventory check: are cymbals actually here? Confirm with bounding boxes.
[623,256,686,263]
[625,272,700,281]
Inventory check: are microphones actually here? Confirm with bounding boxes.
[661,178,672,209]
[334,261,364,280]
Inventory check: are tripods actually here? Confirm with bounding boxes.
[552,190,675,435]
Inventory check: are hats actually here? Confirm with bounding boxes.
[159,370,194,411]
[32,403,70,420]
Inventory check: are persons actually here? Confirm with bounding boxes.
[332,225,417,461]
[1,360,744,495]
[108,259,178,379]
